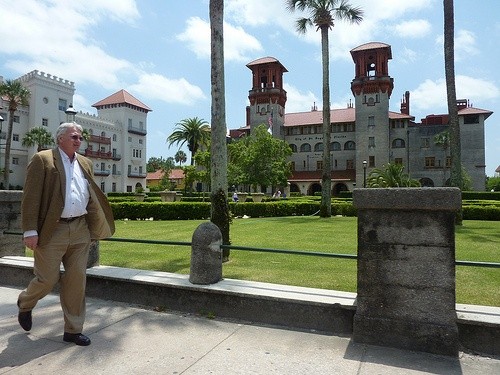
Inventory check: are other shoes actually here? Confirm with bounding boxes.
[17,297,32,331]
[63,332,91,346]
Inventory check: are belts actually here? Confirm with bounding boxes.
[59,217,80,222]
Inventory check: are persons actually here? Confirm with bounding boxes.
[234,190,239,201]
[18,121,115,346]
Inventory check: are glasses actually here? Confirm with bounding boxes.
[62,135,84,141]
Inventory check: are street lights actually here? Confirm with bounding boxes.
[64,103,78,123]
[183,166,188,194]
[362,160,367,188]
